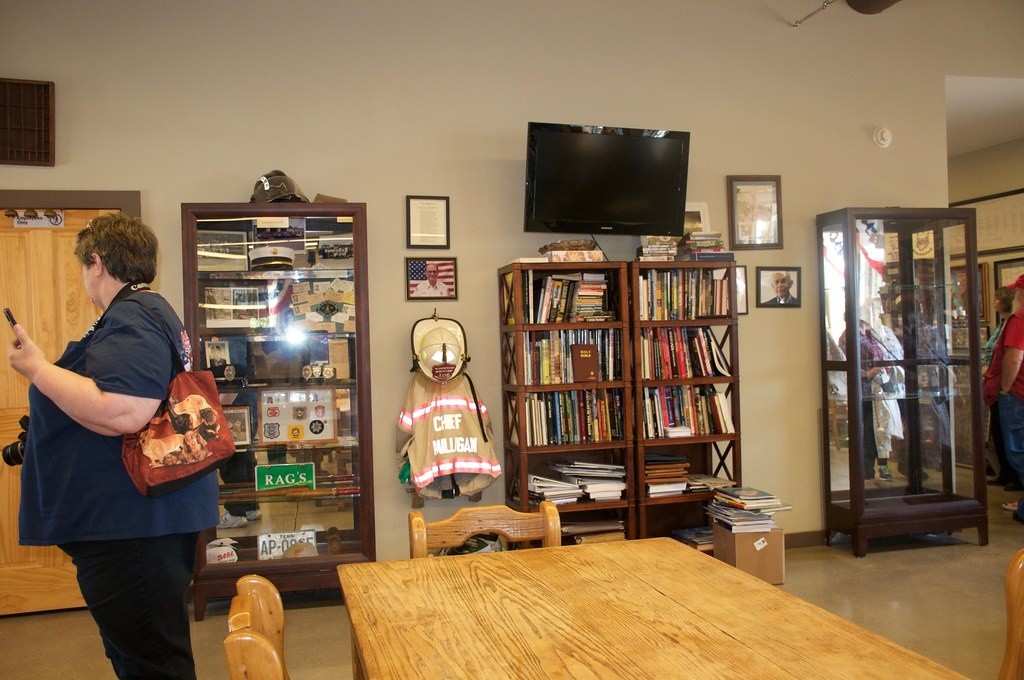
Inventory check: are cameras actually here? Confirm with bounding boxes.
[2,415,29,465]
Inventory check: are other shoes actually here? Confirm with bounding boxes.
[987,478,1024,491]
[1013,512,1024,522]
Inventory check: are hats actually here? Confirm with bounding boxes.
[1007,273,1024,290]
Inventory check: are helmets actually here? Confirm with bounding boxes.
[410,308,471,384]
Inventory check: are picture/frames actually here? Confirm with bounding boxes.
[222,404,253,448]
[949,262,990,323]
[952,326,990,349]
[197,229,249,272]
[736,265,748,315]
[726,175,783,250]
[257,385,338,445]
[994,257,1024,328]
[406,258,458,300]
[205,340,231,368]
[640,202,711,247]
[756,266,802,308]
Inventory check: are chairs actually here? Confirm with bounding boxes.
[999,549,1024,680]
[223,573,291,680]
[408,501,561,558]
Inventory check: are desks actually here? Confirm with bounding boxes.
[337,537,972,680]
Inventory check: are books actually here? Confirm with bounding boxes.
[636,232,735,262]
[504,268,791,553]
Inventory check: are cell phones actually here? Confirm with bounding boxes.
[3,308,18,330]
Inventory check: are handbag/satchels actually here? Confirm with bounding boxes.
[121,371,237,498]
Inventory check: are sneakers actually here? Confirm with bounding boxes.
[879,465,894,481]
[1003,501,1018,510]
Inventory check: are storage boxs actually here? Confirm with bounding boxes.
[712,522,785,585]
[511,250,603,264]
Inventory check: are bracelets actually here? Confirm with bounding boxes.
[1000,388,1009,394]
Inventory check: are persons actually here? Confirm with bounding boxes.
[9,211,220,680]
[414,263,450,297]
[983,274,1024,524]
[212,334,262,528]
[840,274,959,482]
[761,271,798,305]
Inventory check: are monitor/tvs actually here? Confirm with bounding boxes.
[523,122,691,237]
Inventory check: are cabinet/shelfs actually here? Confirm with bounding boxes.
[497,261,742,548]
[180,202,376,621]
